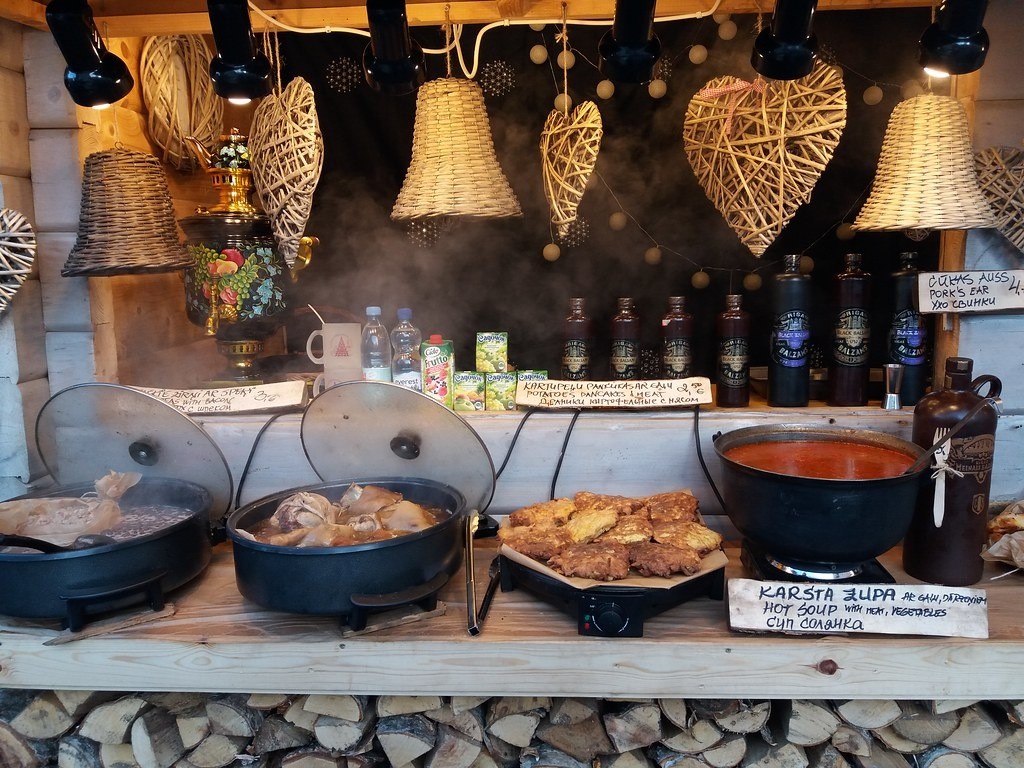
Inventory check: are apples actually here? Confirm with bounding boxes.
[454,349,516,411]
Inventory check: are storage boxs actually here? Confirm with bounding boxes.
[452,331,548,411]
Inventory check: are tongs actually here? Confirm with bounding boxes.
[466,509,503,636]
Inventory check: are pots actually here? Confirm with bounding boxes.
[712,423,932,585]
[493,541,725,638]
[0,477,227,634]
[228,476,468,631]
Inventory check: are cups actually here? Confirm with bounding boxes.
[882,364,905,410]
[307,323,364,397]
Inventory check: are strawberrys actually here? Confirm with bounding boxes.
[427,369,447,396]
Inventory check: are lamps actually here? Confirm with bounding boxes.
[45,0,134,107]
[916,0,990,75]
[362,0,427,98]
[206,0,274,99]
[598,0,661,86]
[750,0,820,80]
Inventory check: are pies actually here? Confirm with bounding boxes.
[498,491,724,581]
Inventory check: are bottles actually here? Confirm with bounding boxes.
[610,298,640,380]
[768,255,811,407]
[717,295,751,408]
[830,254,871,406]
[663,297,692,380]
[178,127,319,388]
[361,306,392,384]
[562,298,593,381]
[892,252,928,406]
[391,308,421,394]
[904,357,1002,587]
